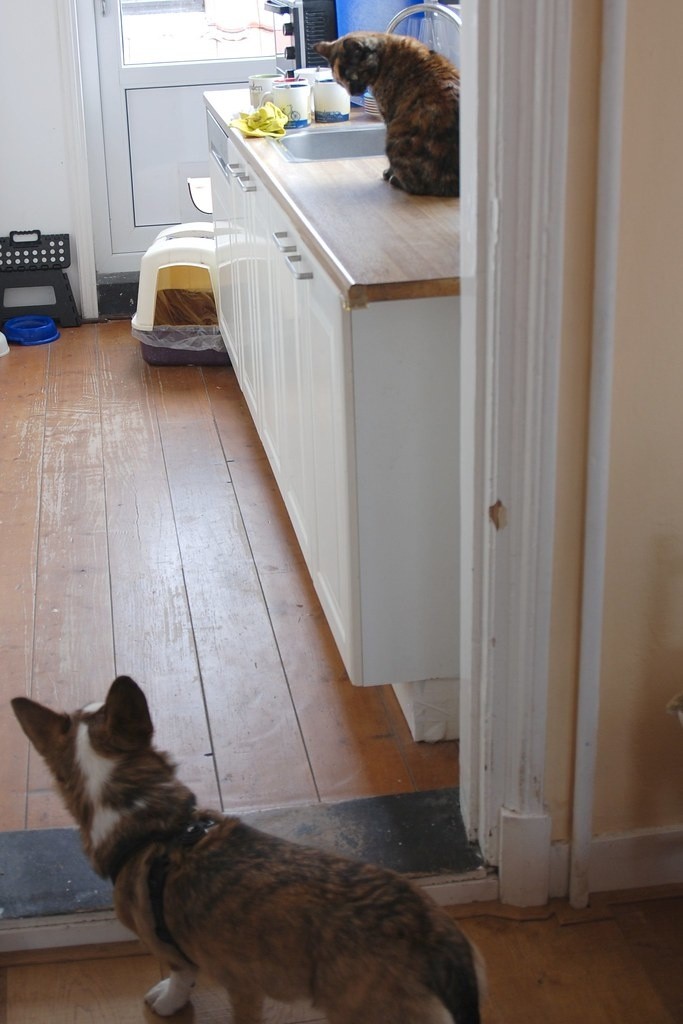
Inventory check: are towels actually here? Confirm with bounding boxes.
[225,100,290,139]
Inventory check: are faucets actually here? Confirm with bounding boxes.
[382,2,463,38]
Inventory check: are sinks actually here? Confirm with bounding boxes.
[263,124,389,165]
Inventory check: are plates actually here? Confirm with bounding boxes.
[363,92,384,121]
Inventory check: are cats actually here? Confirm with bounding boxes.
[314,29,459,198]
[10,674,481,1024]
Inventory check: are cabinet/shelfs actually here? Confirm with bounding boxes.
[205,105,460,686]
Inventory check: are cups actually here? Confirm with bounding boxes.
[313,78,350,123]
[248,74,284,108]
[257,84,312,129]
[294,67,335,111]
[406,18,446,56]
[272,78,308,88]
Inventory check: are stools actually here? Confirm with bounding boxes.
[0,229,82,327]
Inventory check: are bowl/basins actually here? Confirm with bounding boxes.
[2,315,60,346]
[0,331,11,357]
